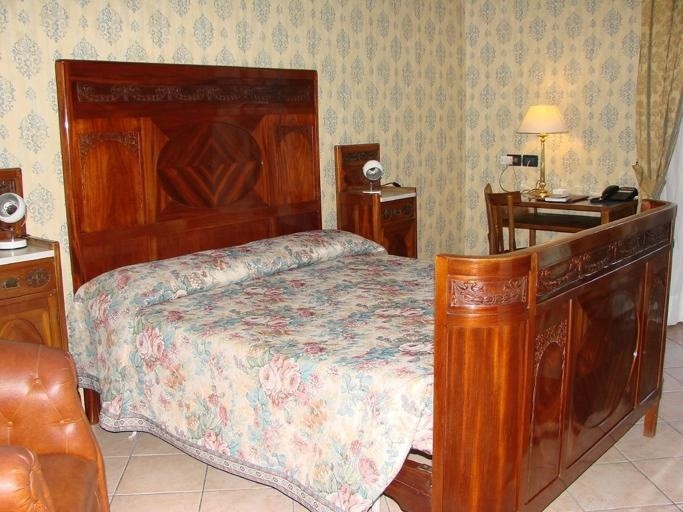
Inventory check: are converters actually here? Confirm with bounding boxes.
[500,156,513,166]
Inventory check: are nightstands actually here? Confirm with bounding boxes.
[0,168,69,352]
[334,143,418,258]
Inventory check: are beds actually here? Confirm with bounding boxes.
[54,57,678,512]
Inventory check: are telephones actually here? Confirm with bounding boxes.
[602,185,638,202]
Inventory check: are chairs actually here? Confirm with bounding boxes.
[0,336,109,511]
[485,184,516,255]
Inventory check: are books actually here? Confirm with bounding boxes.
[544,192,590,202]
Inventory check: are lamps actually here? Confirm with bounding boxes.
[0,192,29,251]
[517,105,568,200]
[362,159,384,196]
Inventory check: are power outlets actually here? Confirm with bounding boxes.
[505,154,521,167]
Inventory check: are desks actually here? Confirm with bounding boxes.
[499,194,638,246]
